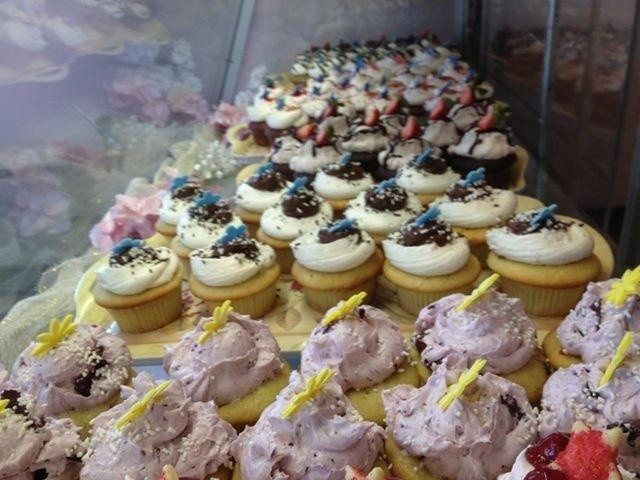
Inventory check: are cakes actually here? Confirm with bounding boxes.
[431,167,546,270]
[7,314,138,440]
[153,172,227,242]
[295,292,420,427]
[535,332,640,440]
[187,235,281,319]
[380,355,537,479]
[485,204,602,318]
[172,190,252,281]
[497,423,637,480]
[161,300,292,425]
[382,205,482,318]
[1,385,87,479]
[341,178,430,253]
[288,216,383,315]
[75,371,237,479]
[254,176,334,273]
[405,273,546,401]
[541,267,640,373]
[235,163,298,234]
[90,236,183,335]
[393,146,460,206]
[309,152,373,221]
[230,367,391,479]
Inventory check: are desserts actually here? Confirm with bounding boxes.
[212,31,518,170]
